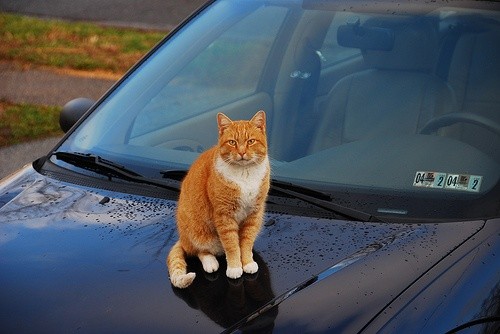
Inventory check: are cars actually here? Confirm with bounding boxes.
[0,1,499,333]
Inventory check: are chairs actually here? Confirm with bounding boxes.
[307,13,500,170]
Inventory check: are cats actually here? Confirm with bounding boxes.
[165,108,271,288]
